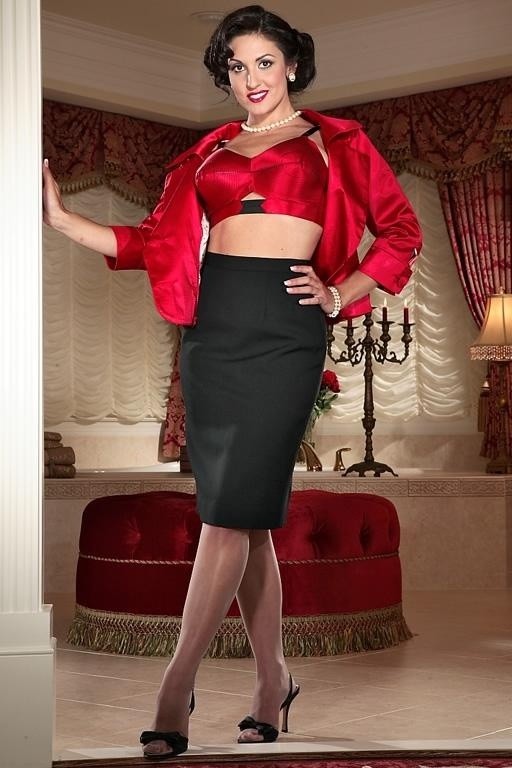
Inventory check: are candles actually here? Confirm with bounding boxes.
[377,298,420,325]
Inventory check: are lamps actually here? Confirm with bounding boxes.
[468,285,512,475]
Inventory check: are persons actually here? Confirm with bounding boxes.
[40,4,427,759]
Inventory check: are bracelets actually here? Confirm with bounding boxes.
[327,285,342,319]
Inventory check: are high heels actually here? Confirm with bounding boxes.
[138,689,196,762]
[239,673,301,745]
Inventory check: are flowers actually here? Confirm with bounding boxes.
[303,370,340,440]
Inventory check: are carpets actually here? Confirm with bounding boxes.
[53,748,511,768]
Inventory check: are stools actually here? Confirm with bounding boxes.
[75,489,406,656]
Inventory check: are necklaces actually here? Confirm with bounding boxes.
[240,109,302,132]
